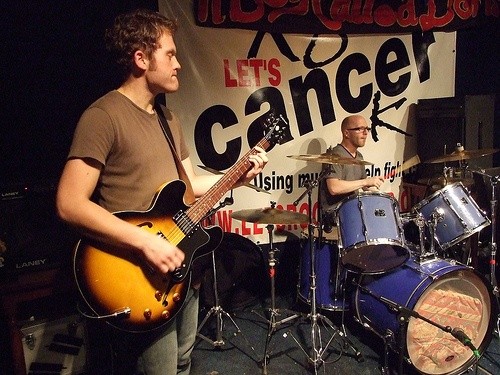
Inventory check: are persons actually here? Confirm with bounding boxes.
[315,115,385,284]
[55,13,268,375]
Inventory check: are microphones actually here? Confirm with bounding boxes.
[452,328,477,355]
[113,308,131,317]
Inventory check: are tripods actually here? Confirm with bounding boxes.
[251,224,304,374]
[194,206,243,348]
[295,163,364,375]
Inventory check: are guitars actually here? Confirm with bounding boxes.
[71,112,288,334]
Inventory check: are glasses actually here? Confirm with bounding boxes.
[347,126,371,133]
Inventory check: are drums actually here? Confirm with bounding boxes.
[353,243,499,375]
[399,211,472,266]
[410,181,491,252]
[296,225,352,312]
[334,190,411,276]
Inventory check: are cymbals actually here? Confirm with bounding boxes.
[196,164,271,194]
[419,148,499,163]
[415,175,475,186]
[286,152,375,165]
[231,207,309,225]
